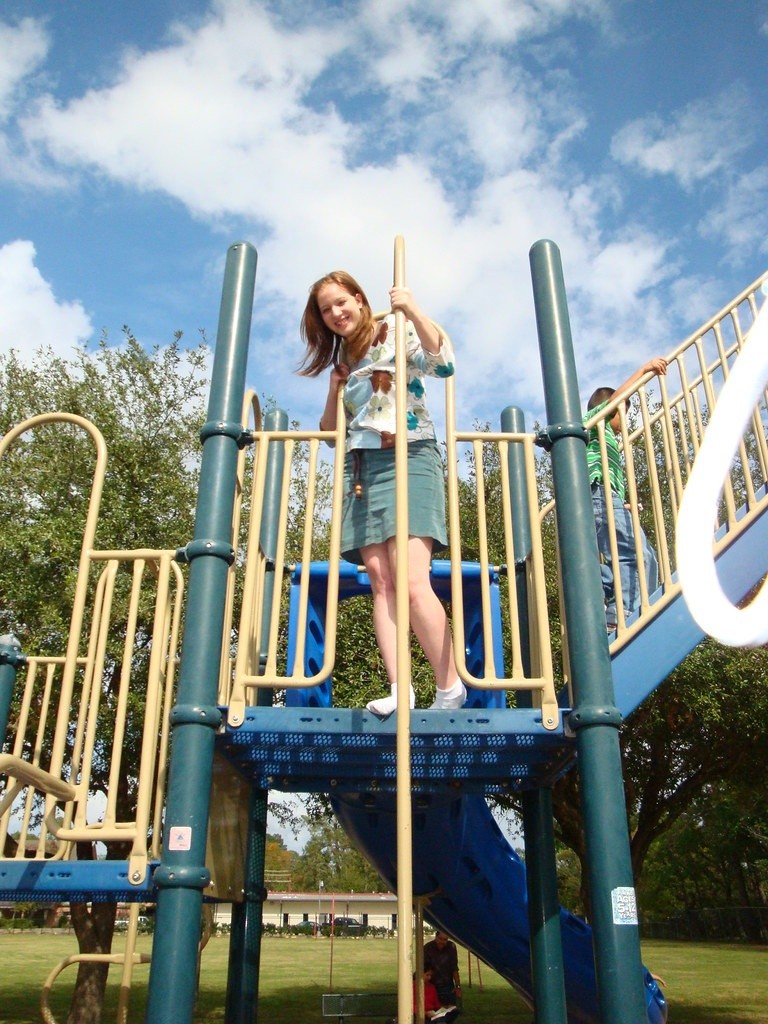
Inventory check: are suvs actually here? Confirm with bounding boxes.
[334,917,363,929]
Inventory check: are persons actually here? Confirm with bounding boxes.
[413,965,457,1023]
[291,271,467,717]
[651,973,667,988]
[423,930,464,1019]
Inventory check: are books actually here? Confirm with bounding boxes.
[430,1004,456,1021]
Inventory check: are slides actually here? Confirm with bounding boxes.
[328,782,671,1024]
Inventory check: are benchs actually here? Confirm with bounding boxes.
[321,991,398,1024]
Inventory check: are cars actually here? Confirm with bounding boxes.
[295,920,323,931]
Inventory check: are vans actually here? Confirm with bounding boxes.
[138,916,150,926]
[114,920,130,925]
